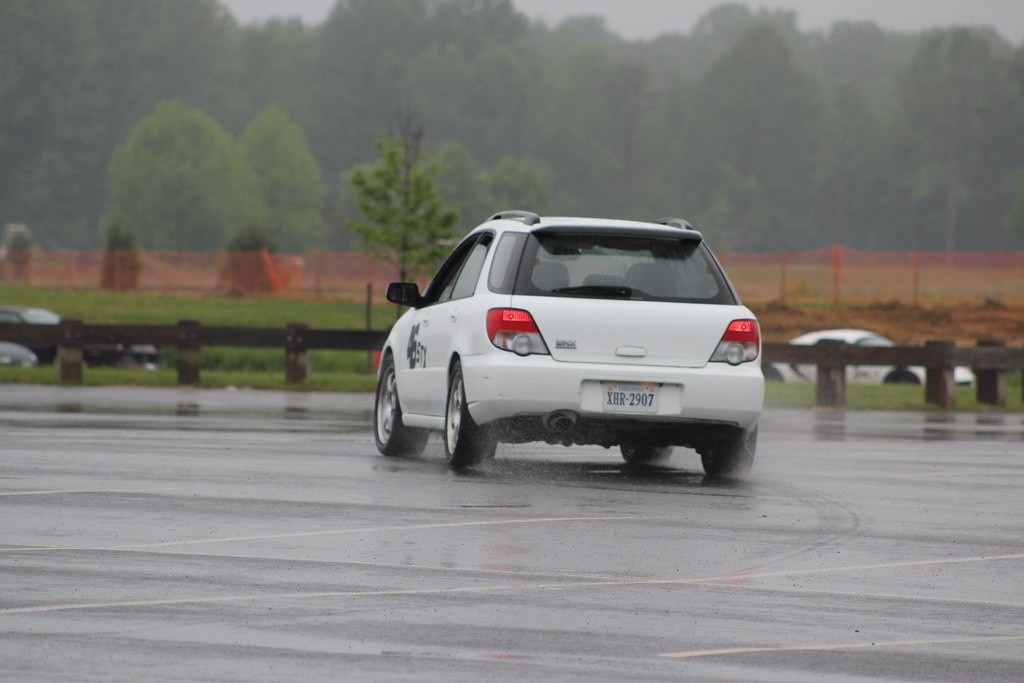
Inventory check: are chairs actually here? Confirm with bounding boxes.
[531,260,570,292]
[622,262,687,298]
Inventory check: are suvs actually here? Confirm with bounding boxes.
[373,209,766,480]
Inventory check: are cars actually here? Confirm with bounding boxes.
[1,306,158,372]
[768,327,977,389]
